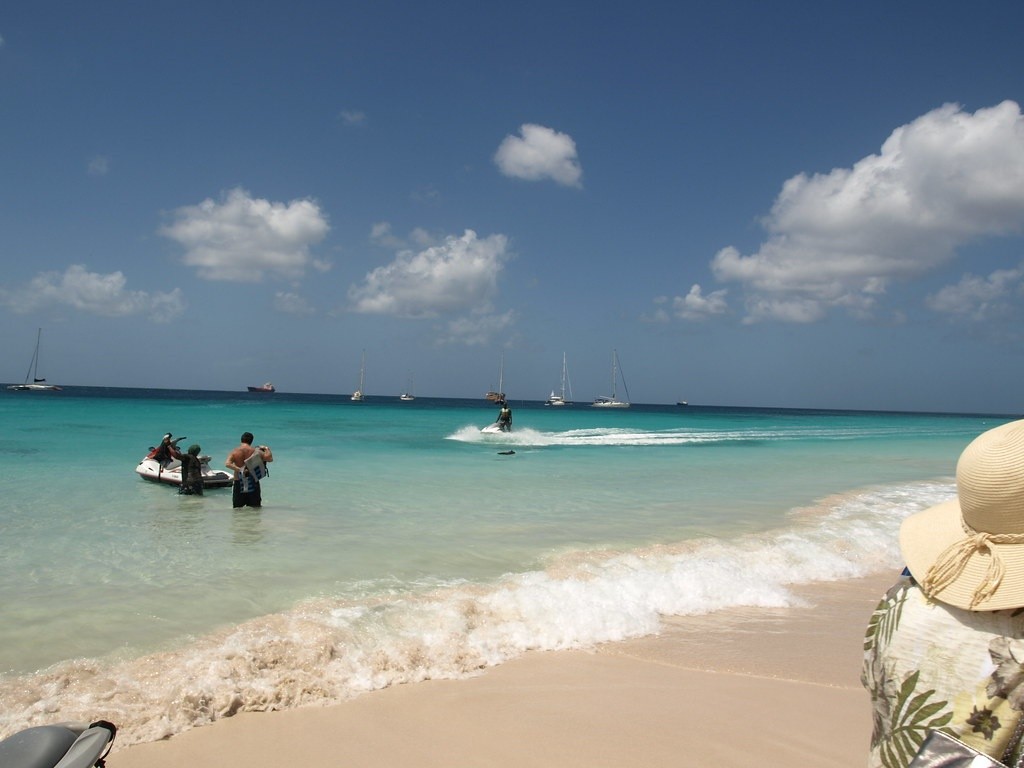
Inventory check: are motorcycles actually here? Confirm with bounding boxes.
[136,437,236,487]
[480,422,505,435]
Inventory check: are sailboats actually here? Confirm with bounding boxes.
[590,348,632,408]
[542,352,573,405]
[351,348,366,401]
[494,351,507,404]
[8,324,62,391]
[400,363,417,401]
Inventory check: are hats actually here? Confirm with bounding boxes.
[900,418,1024,611]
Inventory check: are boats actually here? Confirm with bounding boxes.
[485,392,498,400]
[247,383,275,393]
[677,400,688,406]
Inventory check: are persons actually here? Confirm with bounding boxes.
[225,431,273,508]
[862,415,1023,767]
[163,436,204,496]
[496,402,512,432]
